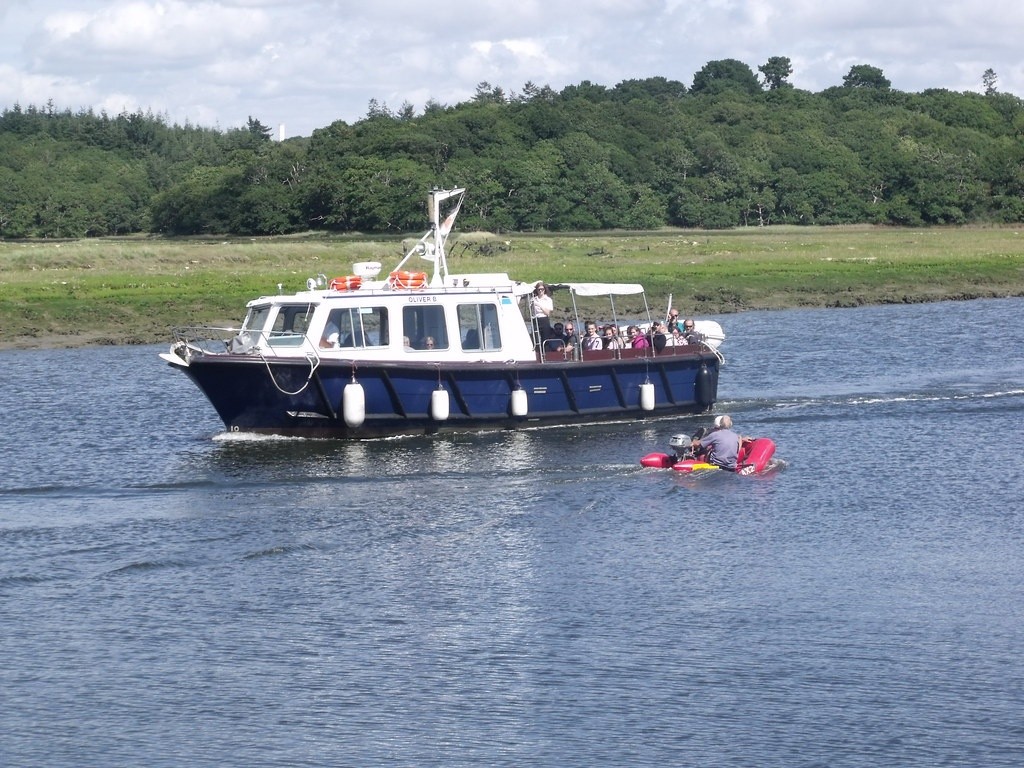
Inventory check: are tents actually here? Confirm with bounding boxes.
[519,281,655,364]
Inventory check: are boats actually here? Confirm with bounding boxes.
[639,436,776,476]
[155,184,728,441]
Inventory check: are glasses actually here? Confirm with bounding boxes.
[555,327,562,330]
[566,329,572,330]
[686,326,692,328]
[426,344,433,346]
[537,288,544,291]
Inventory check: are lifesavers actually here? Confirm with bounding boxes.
[331,275,361,290]
[391,270,427,289]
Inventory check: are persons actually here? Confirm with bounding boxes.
[549,309,701,353]
[694,415,757,473]
[317,320,340,349]
[463,329,479,350]
[402,336,415,352]
[528,282,552,347]
[420,335,437,350]
